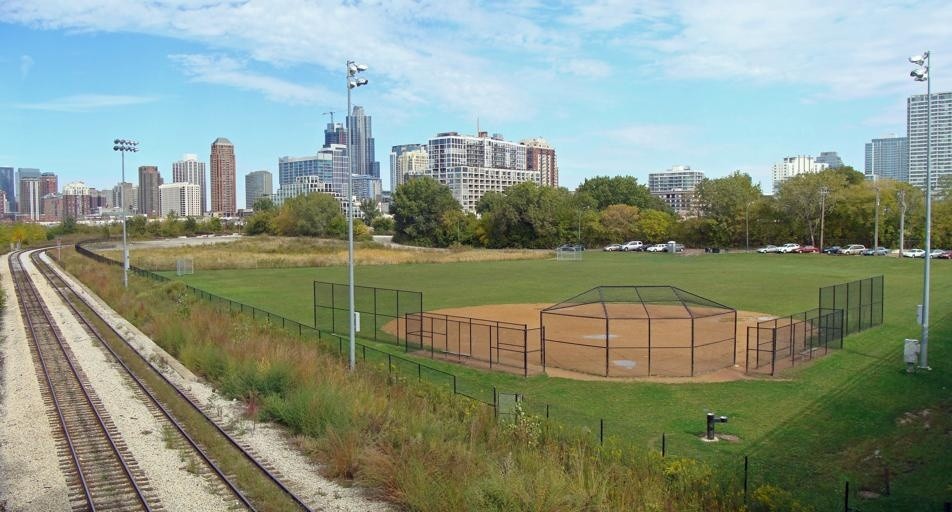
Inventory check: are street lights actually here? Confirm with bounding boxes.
[907,48,936,374]
[113,139,141,291]
[577,205,591,241]
[815,182,831,254]
[895,187,906,257]
[346,58,369,377]
[871,184,884,250]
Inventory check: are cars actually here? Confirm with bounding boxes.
[554,242,588,251]
[601,240,686,253]
[755,242,952,260]
